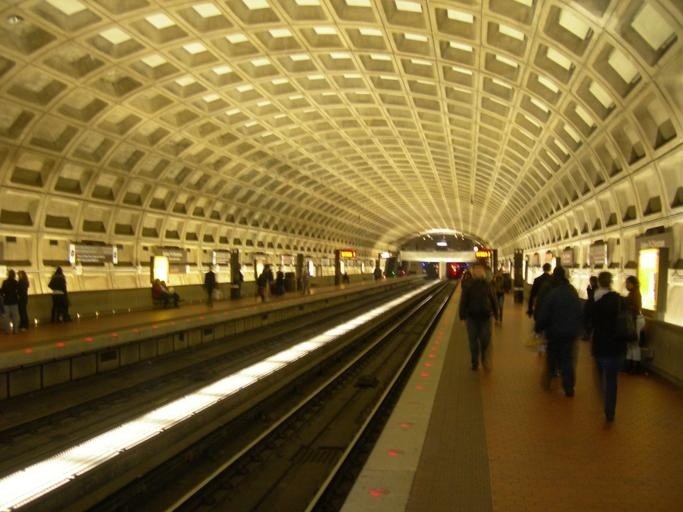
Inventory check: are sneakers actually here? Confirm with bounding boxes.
[472,361,493,370]
[565,385,575,397]
[604,411,615,421]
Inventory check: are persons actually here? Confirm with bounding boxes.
[48,267,72,323]
[374,267,382,280]
[152,279,184,308]
[0,270,29,335]
[205,266,311,308]
[459,262,646,422]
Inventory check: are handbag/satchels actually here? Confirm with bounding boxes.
[617,295,637,342]
